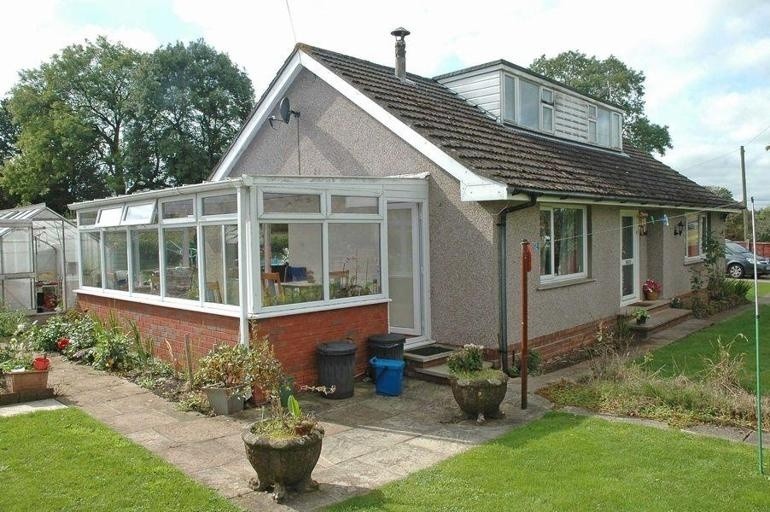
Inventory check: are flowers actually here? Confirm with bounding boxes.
[643,279,663,294]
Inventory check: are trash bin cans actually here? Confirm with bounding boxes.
[368,331,407,379]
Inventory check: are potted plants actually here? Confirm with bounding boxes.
[0,342,54,394]
[442,342,509,425]
[195,313,325,505]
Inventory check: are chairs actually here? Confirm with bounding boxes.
[261,271,282,297]
[206,281,224,303]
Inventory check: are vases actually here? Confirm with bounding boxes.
[646,290,658,301]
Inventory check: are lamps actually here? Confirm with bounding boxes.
[674,220,685,237]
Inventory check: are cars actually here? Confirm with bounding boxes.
[724,239,770,278]
